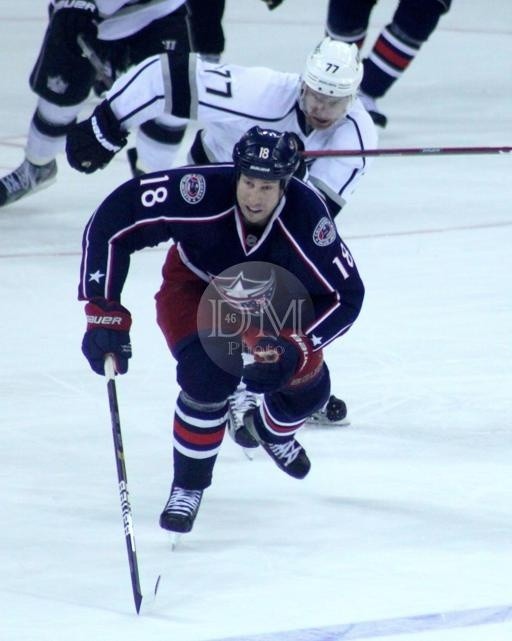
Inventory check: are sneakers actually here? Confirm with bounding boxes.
[359,91,386,128]
[0,158,57,205]
[161,390,346,533]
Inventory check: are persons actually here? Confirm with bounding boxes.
[65,32,380,449]
[0,1,227,210]
[323,0,452,130]
[75,126,364,536]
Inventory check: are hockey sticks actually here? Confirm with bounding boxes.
[103,356,162,619]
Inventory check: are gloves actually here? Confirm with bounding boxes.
[242,333,312,393]
[52,8,98,63]
[67,101,128,173]
[82,299,131,375]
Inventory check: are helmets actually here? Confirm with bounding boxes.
[232,126,301,180]
[301,37,364,97]
